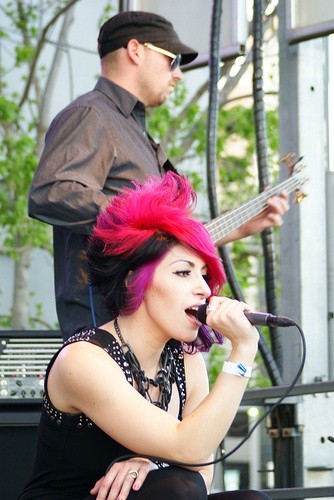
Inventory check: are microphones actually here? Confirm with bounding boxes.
[198,304,295,327]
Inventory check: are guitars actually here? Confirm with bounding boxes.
[200,150,310,243]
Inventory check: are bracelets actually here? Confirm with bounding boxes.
[221,358,254,379]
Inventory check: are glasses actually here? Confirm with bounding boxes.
[144,42,181,69]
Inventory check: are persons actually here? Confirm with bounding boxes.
[14,171,260,500]
[28,10,291,355]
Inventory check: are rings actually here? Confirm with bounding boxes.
[130,468,139,480]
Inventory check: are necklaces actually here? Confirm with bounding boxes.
[113,317,174,411]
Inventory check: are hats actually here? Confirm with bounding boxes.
[98,11,198,65]
